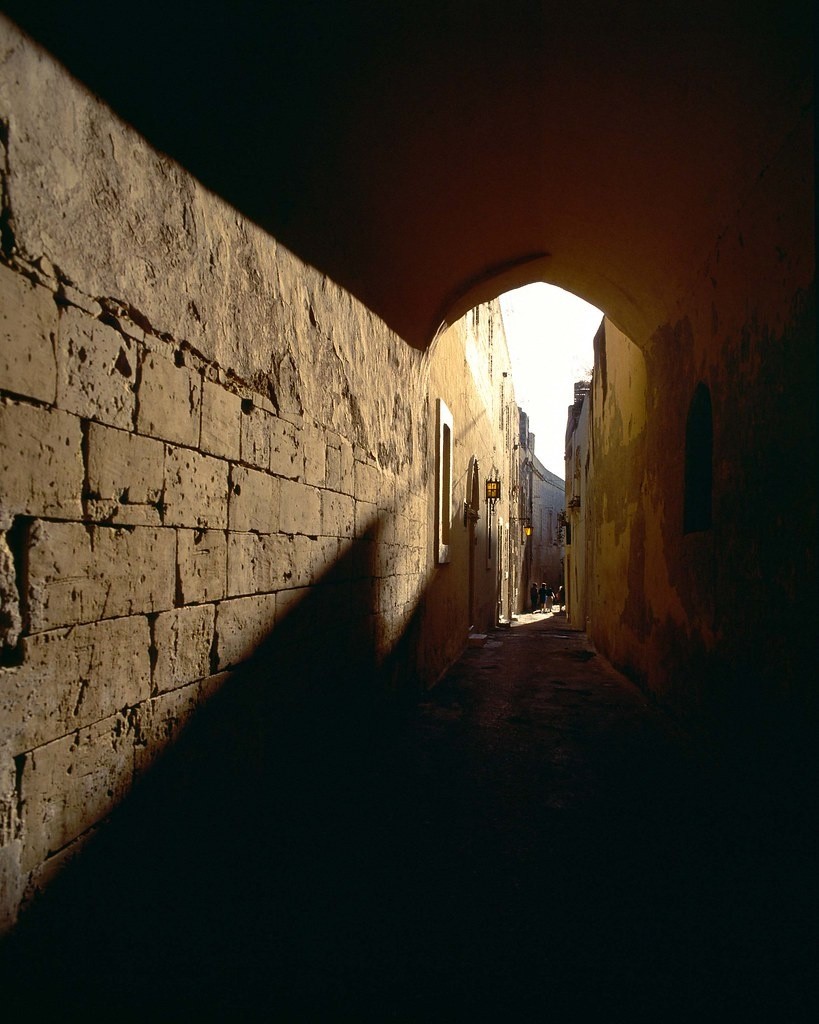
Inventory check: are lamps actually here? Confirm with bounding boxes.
[486,478,502,513]
[519,517,534,536]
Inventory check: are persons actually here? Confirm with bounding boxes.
[531,583,555,613]
[558,587,565,612]
